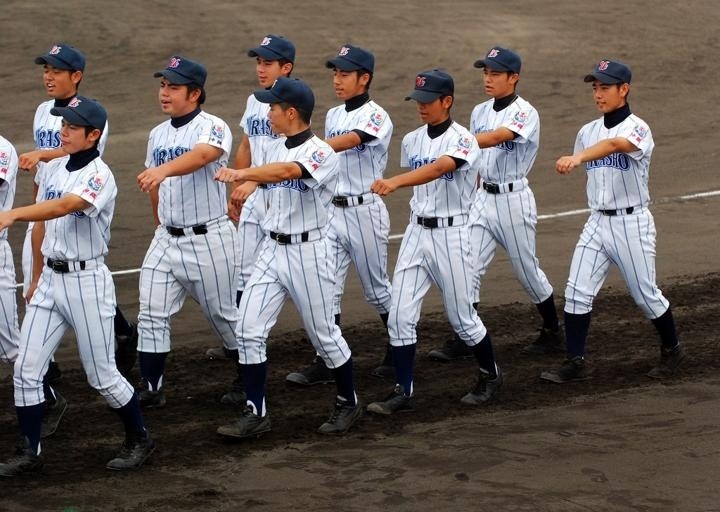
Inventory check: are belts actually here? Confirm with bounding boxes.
[271,231,308,245]
[167,225,205,237]
[47,257,85,273]
[333,195,362,206]
[484,181,513,193]
[600,208,633,215]
[418,216,453,228]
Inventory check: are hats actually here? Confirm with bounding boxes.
[50,96,105,129]
[35,44,85,70]
[249,35,294,61]
[584,59,632,84]
[254,76,314,108]
[405,69,454,103]
[326,46,374,73]
[473,47,522,74]
[153,56,207,86]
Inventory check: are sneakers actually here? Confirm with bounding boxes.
[540,357,585,383]
[218,413,270,436]
[221,388,248,406]
[206,347,231,360]
[140,389,165,408]
[428,348,474,362]
[523,326,564,354]
[462,367,503,406]
[647,352,694,378]
[39,391,68,437]
[107,428,155,470]
[286,356,416,436]
[1,437,43,476]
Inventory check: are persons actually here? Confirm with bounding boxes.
[213,74,363,441]
[284,42,402,391]
[17,42,139,386]
[0,133,70,442]
[3,92,161,480]
[534,55,685,389]
[126,51,247,409]
[424,45,562,364]
[204,33,297,363]
[362,65,504,418]
